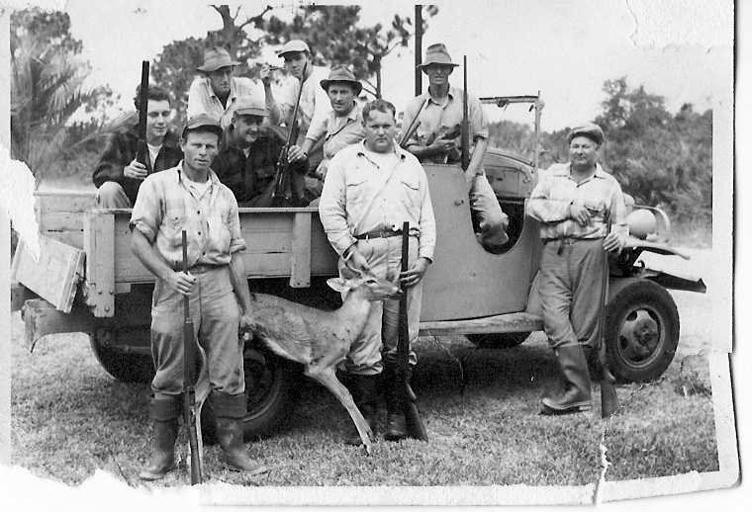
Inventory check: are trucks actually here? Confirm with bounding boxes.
[8,63,683,436]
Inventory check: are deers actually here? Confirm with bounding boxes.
[185,233,404,484]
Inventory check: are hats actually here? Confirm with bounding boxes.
[319,66,363,97]
[566,122,605,151]
[180,114,226,140]
[278,38,311,58]
[197,46,241,74]
[415,41,461,71]
[231,94,270,119]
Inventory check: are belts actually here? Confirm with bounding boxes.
[544,236,583,256]
[357,230,402,240]
[177,264,219,273]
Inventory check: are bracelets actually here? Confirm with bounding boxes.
[303,152,309,156]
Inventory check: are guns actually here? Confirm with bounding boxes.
[397,221,428,442]
[182,230,203,486]
[596,218,620,418]
[126,61,151,207]
[267,63,307,207]
[461,55,470,171]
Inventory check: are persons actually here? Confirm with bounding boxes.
[130,113,267,480]
[301,65,368,207]
[317,100,438,444]
[209,98,311,206]
[91,85,185,210]
[398,42,512,253]
[187,45,265,127]
[524,123,630,415]
[262,39,333,175]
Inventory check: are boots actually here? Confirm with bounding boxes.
[540,344,595,416]
[384,366,413,439]
[344,372,382,446]
[212,392,268,476]
[139,393,182,480]
